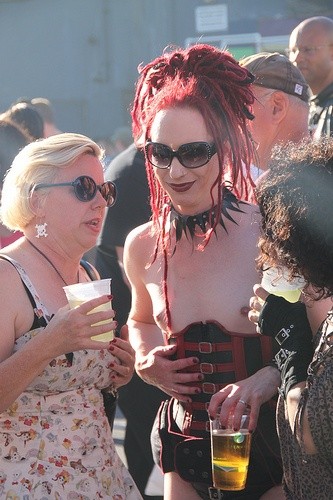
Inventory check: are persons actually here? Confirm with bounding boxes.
[0,132,145,500]
[30,97,63,139]
[1,102,44,140]
[246,135,333,500]
[94,140,172,500]
[238,51,311,187]
[123,42,313,500]
[0,118,34,251]
[288,16,333,138]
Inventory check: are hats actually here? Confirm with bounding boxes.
[239,51,309,101]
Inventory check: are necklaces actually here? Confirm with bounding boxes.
[23,235,80,286]
[160,180,252,258]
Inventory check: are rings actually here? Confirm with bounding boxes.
[238,400,248,408]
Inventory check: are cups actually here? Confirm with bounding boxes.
[62,278,115,342]
[209,404,252,491]
[254,262,307,326]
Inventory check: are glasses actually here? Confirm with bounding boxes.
[144,141,217,169]
[29,175,118,207]
